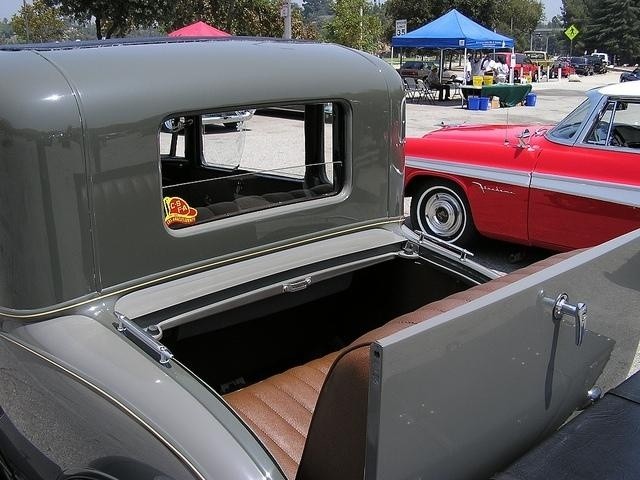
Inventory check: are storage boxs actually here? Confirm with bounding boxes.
[527,94,536,106]
[479,97,489,110]
[468,98,479,110]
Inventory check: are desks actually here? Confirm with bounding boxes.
[458,84,532,108]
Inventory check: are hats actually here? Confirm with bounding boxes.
[431,65,438,71]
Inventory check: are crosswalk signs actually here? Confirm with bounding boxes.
[563,23,581,40]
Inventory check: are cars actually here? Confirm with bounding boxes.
[261,101,336,124]
[0,37,639,478]
[403,79,640,259]
[396,48,640,89]
[165,106,258,135]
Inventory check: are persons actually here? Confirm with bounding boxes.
[465,49,509,85]
[428,65,452,101]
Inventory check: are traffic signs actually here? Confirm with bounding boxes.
[395,19,408,39]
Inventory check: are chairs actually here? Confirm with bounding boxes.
[404,77,466,105]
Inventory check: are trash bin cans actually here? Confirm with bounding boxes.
[526,93,536,106]
[468,97,490,110]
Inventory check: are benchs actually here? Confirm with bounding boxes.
[219,229,639,479]
